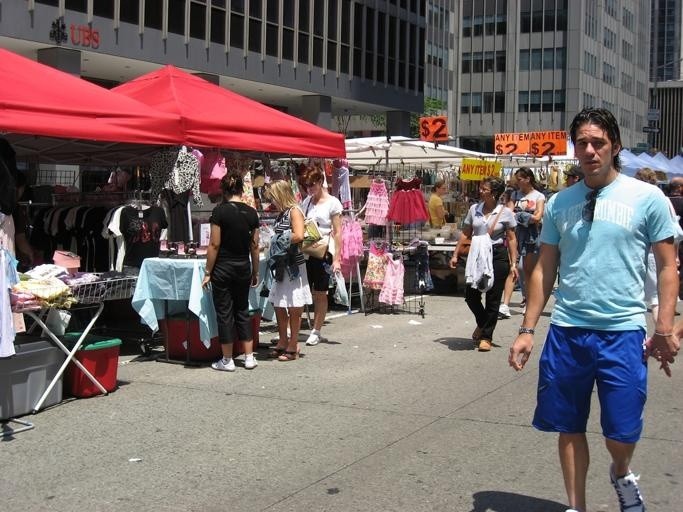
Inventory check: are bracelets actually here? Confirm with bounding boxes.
[250,269,259,276]
[509,262,516,269]
[517,326,535,337]
[652,330,673,339]
[204,270,210,276]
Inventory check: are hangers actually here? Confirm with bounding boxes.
[54,189,151,208]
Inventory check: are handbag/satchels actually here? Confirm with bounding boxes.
[283,217,329,260]
[457,239,471,257]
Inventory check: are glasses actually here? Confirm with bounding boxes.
[582,189,598,222]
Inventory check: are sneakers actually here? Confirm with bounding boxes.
[272,335,290,344]
[273,346,297,360]
[609,462,648,512]
[245,358,257,369]
[472,304,512,351]
[211,358,235,371]
[306,334,320,345]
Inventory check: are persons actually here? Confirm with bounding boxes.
[634,167,683,336]
[270,165,343,344]
[11,171,35,272]
[506,105,680,511]
[499,168,545,318]
[449,176,519,351]
[200,173,263,373]
[262,179,310,362]
[427,168,584,305]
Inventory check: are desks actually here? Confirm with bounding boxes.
[130,250,313,369]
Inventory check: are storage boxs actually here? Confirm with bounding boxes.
[61,332,123,399]
[0,334,67,420]
[167,308,263,362]
